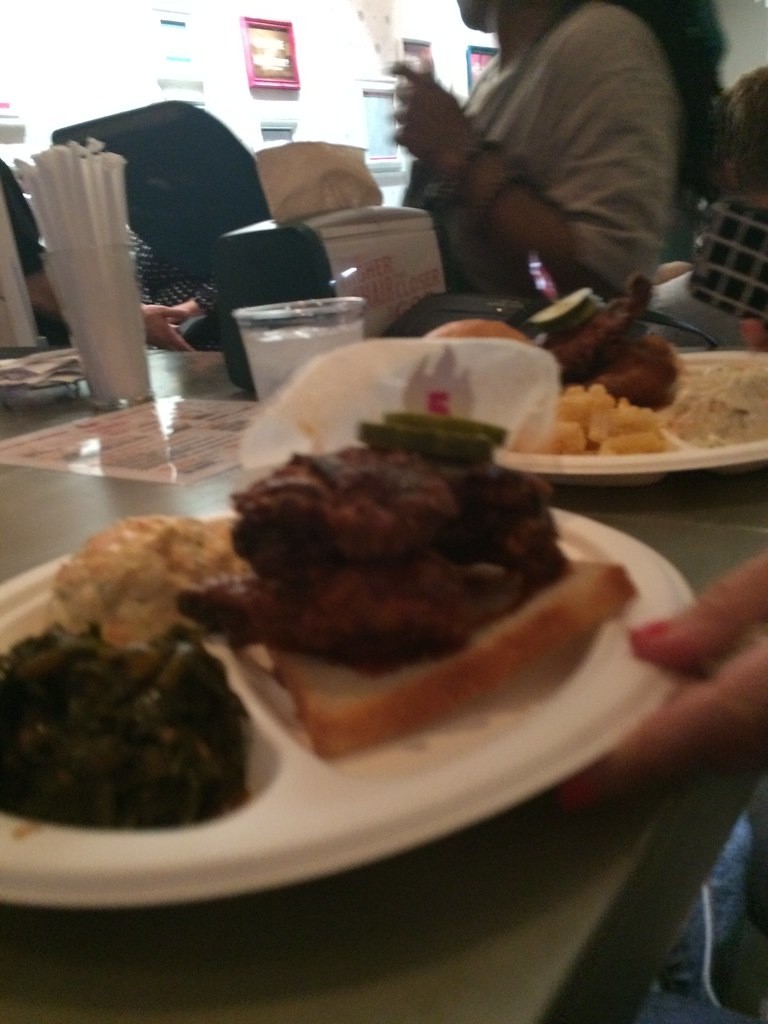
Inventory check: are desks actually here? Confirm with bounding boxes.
[0,344,768,1024]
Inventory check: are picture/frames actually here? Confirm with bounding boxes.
[465,44,498,95]
[352,77,403,168]
[239,15,301,91]
[401,36,436,77]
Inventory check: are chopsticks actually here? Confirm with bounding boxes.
[16,139,128,250]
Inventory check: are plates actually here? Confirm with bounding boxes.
[492,351,768,485]
[0,507,695,905]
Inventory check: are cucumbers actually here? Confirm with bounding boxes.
[358,412,508,462]
[522,288,601,335]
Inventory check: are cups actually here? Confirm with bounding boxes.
[42,242,150,408]
[232,296,365,402]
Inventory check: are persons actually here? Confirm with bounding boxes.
[687,66,768,333]
[0,161,258,401]
[389,0,684,302]
[556,546,768,814]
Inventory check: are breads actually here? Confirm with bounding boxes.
[266,561,641,759]
